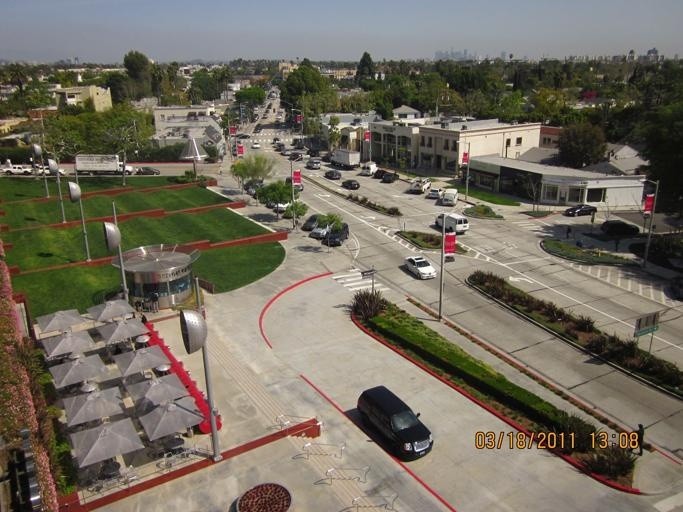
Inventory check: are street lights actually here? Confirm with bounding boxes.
[291,154,306,231]
[437,204,473,322]
[291,109,304,148]
[455,140,471,201]
[359,122,372,163]
[639,178,660,268]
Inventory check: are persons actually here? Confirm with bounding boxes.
[628,423,645,456]
[615,239,620,252]
[566,226,571,238]
[219,165,223,175]
[145,293,159,313]
[113,345,122,363]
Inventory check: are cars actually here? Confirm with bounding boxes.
[565,204,597,217]
[246,175,303,211]
[404,255,437,280]
[291,149,341,180]
[241,101,291,158]
[0,164,65,177]
[342,180,360,190]
[136,167,160,175]
[361,161,459,207]
[301,213,349,247]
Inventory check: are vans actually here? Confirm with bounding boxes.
[357,385,434,461]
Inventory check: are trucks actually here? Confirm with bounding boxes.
[75,153,134,176]
[331,149,361,170]
